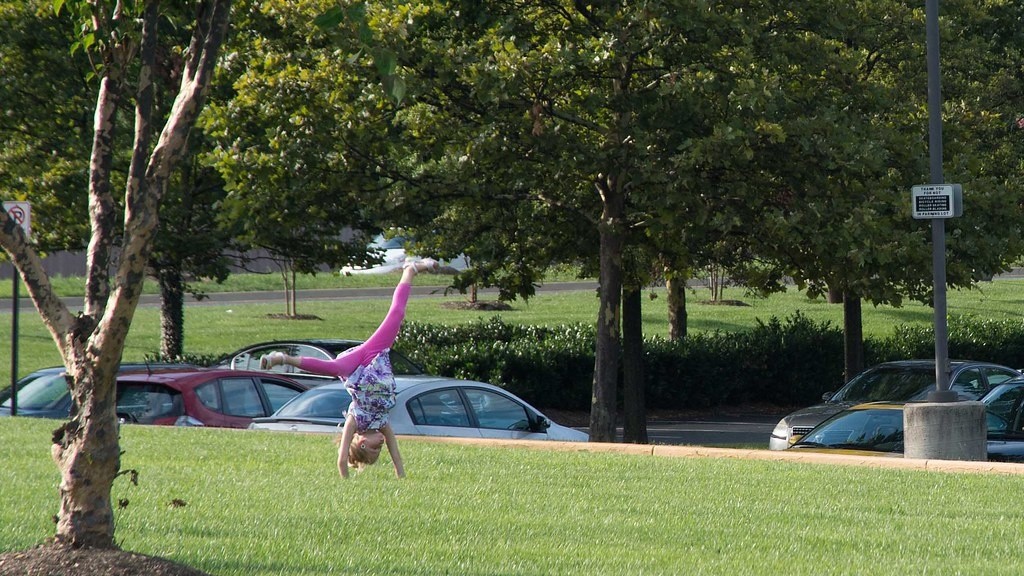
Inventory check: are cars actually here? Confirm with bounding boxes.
[770,358,1024,465]
[2,366,316,430]
[208,340,425,378]
[243,375,589,440]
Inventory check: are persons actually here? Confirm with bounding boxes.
[259,256,440,478]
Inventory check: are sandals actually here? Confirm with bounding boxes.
[260,350,284,370]
[403,257,439,275]
